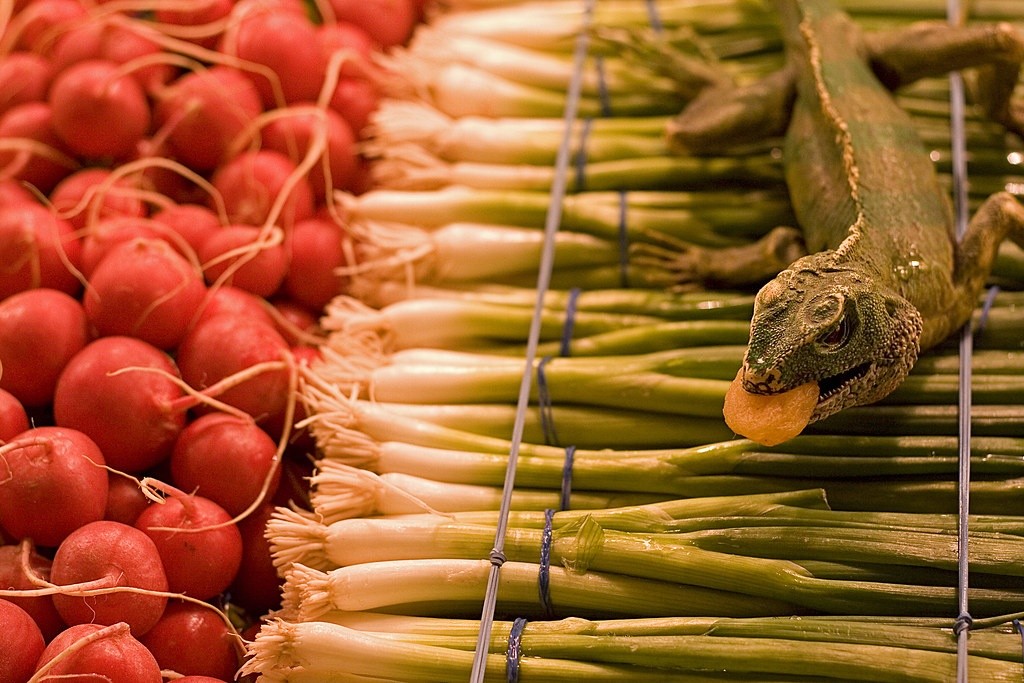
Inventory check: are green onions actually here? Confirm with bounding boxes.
[243,0,1024,683]
